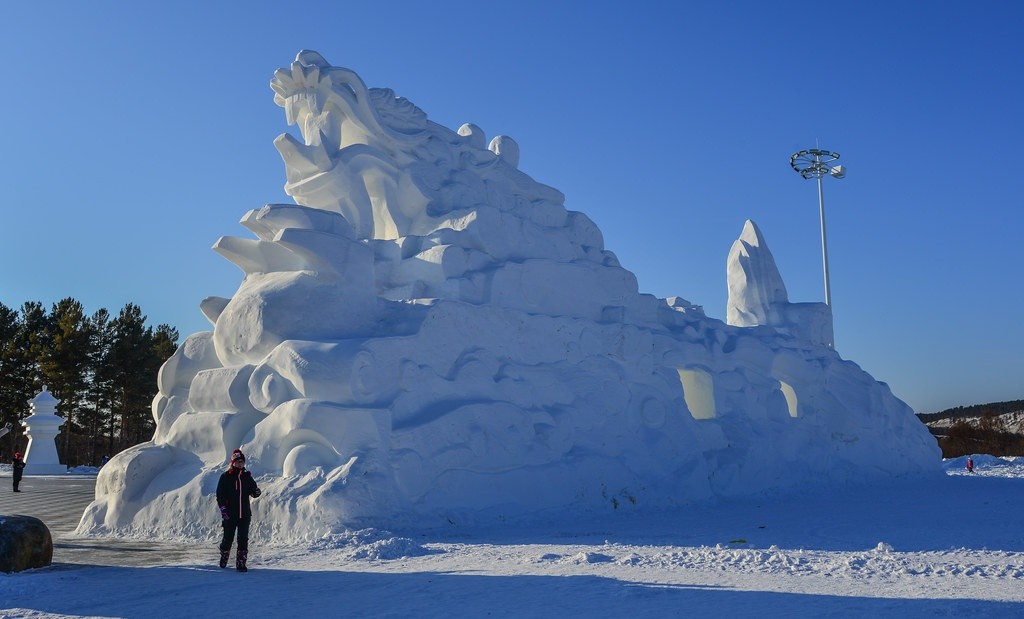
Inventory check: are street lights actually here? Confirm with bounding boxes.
[789,138,847,350]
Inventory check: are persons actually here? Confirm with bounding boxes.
[967,457,973,473]
[100,455,110,465]
[11,452,26,492]
[215,448,261,571]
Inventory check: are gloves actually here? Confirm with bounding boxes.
[221,510,231,521]
[255,488,261,497]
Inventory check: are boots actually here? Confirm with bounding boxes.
[237,549,248,571]
[219,548,230,567]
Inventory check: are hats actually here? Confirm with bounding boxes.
[231,450,245,463]
[15,453,22,459]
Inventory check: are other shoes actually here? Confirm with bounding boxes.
[14,490,20,492]
